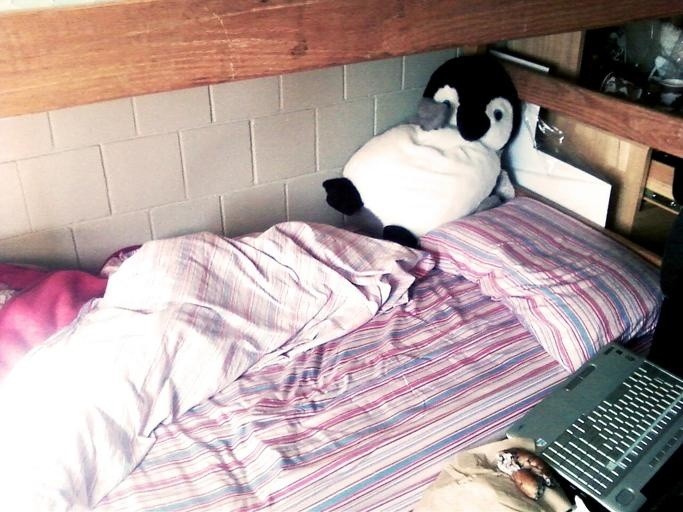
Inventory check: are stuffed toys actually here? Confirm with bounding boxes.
[322,52,525,250]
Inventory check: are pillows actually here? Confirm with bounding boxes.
[418,196,669,375]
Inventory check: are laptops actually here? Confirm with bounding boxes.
[506,342,683,512]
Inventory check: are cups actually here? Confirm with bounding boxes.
[661,77,682,112]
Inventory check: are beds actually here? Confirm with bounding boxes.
[0,53,682,512]
